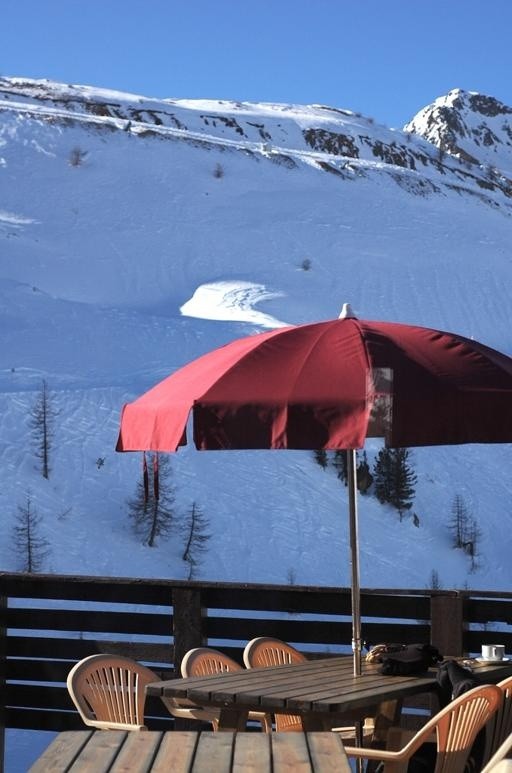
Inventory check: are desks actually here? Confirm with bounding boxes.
[145,655,512,737]
[22,731,350,773]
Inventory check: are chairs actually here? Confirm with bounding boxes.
[178,647,271,737]
[342,686,502,773]
[486,678,512,770]
[243,637,379,744]
[67,654,221,734]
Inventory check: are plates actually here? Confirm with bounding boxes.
[475,657,511,666]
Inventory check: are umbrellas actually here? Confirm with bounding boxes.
[116,299,512,769]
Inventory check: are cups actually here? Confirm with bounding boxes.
[482,644,505,659]
